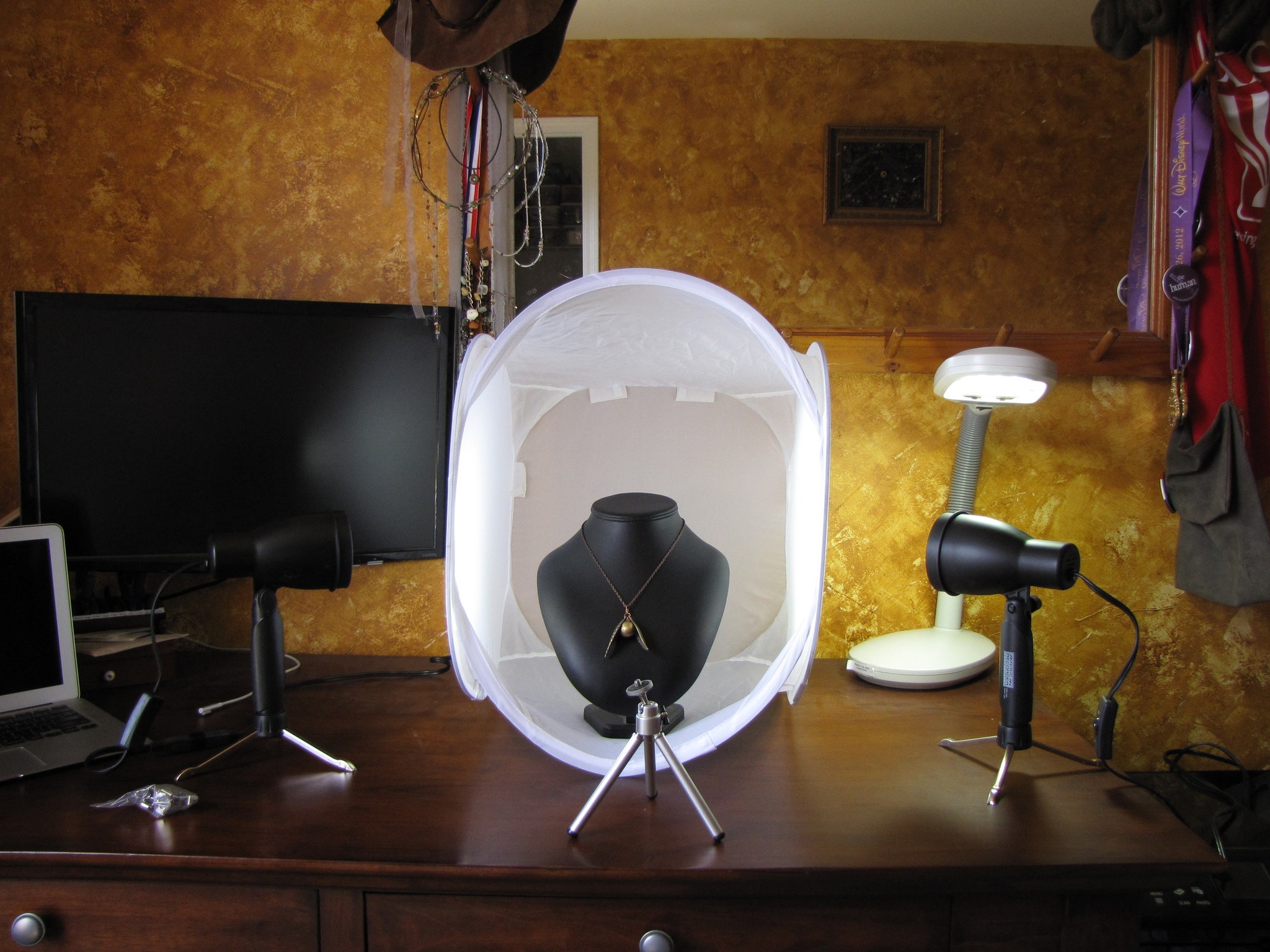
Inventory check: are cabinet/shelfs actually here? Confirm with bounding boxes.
[0,645,1270,952]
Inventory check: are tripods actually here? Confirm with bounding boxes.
[565,675,730,849]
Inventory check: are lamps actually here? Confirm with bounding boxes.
[168,508,362,787]
[921,507,1107,809]
[819,115,949,232]
[845,344,1059,694]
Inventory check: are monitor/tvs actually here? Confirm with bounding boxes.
[13,287,463,578]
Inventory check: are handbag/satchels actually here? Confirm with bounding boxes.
[1159,400,1270,608]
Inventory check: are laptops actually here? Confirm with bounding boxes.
[0,521,128,782]
